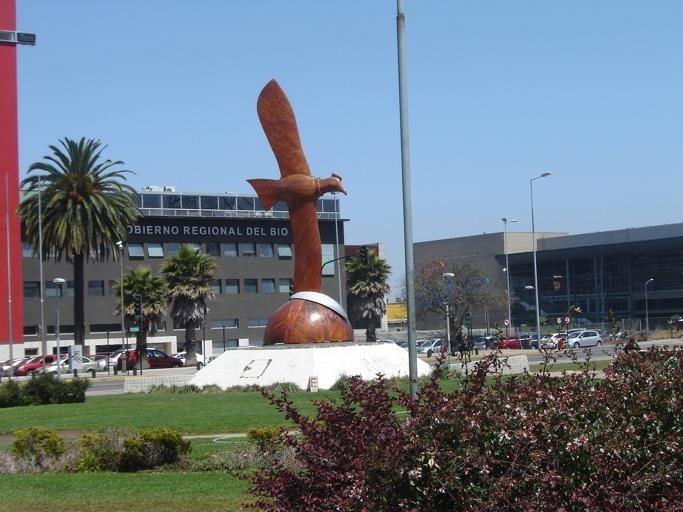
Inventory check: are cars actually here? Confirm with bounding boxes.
[395,327,648,354]
[0,347,205,377]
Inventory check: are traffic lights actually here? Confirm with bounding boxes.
[133,295,142,314]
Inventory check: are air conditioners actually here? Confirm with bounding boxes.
[163,186,175,193]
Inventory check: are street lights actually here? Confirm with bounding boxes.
[53,275,66,378]
[114,239,128,373]
[442,271,455,356]
[501,217,519,339]
[644,277,654,339]
[529,170,552,351]
